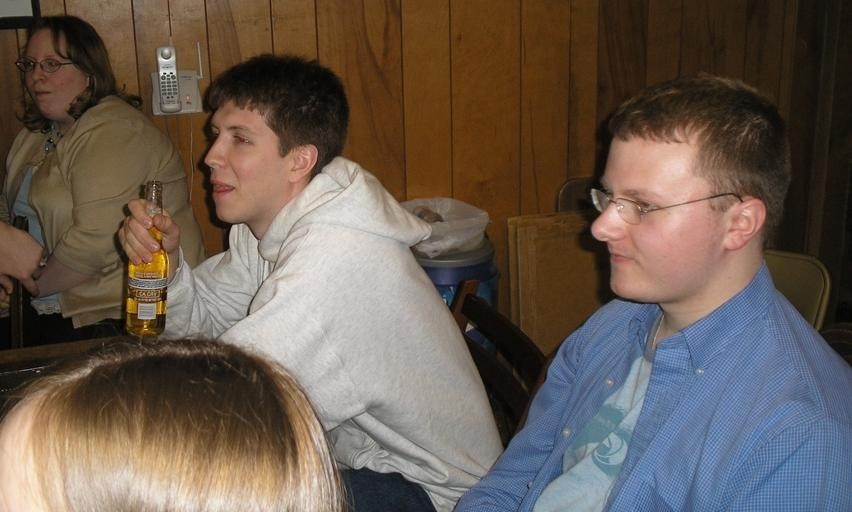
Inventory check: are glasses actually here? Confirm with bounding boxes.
[15,57,74,73]
[591,187,744,225]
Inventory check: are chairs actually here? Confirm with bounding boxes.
[451,279,556,445]
[763,249,831,333]
[9,216,32,349]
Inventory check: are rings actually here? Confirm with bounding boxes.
[40,259,46,266]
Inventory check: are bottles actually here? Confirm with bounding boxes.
[122,179,171,338]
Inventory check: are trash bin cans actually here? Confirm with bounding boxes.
[398,196,502,318]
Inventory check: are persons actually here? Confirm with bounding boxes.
[454,71,852,512]
[118,53,505,512]
[0,221,46,296]
[0,339,355,512]
[0,15,204,349]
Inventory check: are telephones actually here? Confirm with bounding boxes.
[151,37,203,115]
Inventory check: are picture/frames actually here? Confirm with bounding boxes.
[0,0,41,30]
